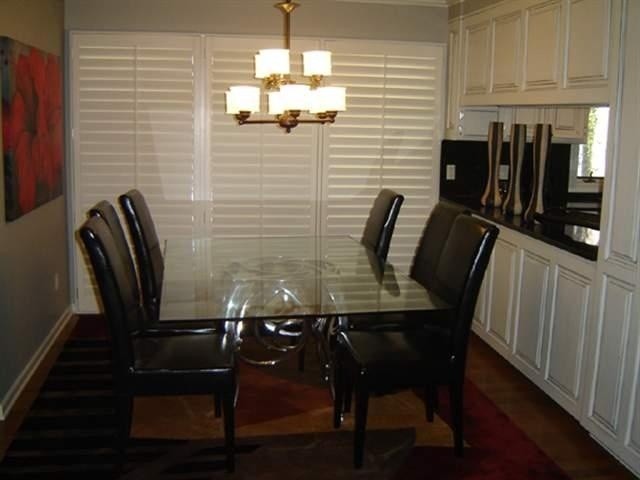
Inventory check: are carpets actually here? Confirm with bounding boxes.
[0,314,570,480]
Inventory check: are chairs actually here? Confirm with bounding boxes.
[79,216,236,473]
[334,213,500,471]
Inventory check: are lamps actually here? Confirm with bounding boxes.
[224,0,346,133]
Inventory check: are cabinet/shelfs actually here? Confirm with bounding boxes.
[498,108,588,144]
